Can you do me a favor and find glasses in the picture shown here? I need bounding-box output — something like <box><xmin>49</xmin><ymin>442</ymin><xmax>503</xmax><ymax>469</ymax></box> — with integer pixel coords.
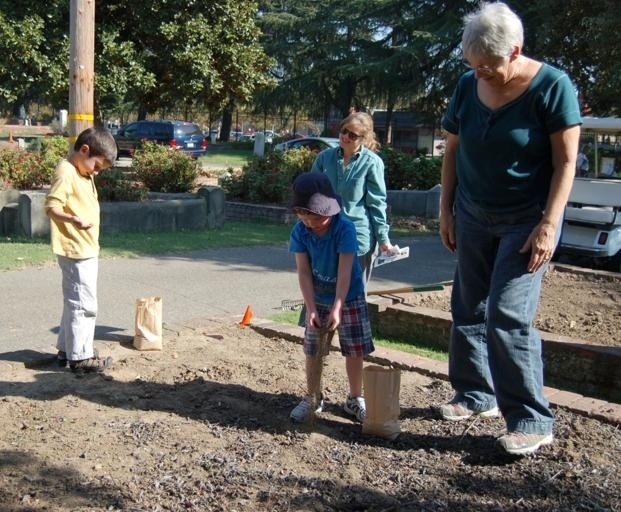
<box><xmin>339</xmin><ymin>127</ymin><xmax>364</xmax><ymax>141</ymax></box>
<box><xmin>461</xmin><ymin>56</ymin><xmax>504</xmax><ymax>74</ymax></box>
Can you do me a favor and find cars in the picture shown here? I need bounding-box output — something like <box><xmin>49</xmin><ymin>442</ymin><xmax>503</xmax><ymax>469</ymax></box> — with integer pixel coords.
<box><xmin>274</xmin><ymin>135</ymin><xmax>339</xmax><ymax>157</ymax></box>
<box><xmin>201</xmin><ymin>127</ymin><xmax>308</xmax><ymax>144</ymax></box>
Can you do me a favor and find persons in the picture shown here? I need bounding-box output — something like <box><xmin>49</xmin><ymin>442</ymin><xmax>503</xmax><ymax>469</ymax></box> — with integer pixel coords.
<box><xmin>438</xmin><ymin>3</ymin><xmax>584</xmax><ymax>458</ymax></box>
<box><xmin>289</xmin><ymin>172</ymin><xmax>375</xmax><ymax>422</ymax></box>
<box><xmin>297</xmin><ymin>112</ymin><xmax>395</xmax><ymax>366</ymax></box>
<box><xmin>45</xmin><ymin>126</ymin><xmax>118</xmax><ymax>373</ymax></box>
<box><xmin>576</xmin><ymin>142</ymin><xmax>592</xmax><ymax>177</ymax></box>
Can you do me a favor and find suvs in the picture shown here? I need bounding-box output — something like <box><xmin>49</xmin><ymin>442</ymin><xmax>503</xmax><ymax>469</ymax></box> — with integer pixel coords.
<box><xmin>112</xmin><ymin>117</ymin><xmax>207</xmax><ymax>164</ymax></box>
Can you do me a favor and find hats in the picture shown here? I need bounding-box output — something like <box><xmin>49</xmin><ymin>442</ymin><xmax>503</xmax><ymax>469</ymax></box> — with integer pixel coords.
<box><xmin>288</xmin><ymin>173</ymin><xmax>341</xmax><ymax>217</ymax></box>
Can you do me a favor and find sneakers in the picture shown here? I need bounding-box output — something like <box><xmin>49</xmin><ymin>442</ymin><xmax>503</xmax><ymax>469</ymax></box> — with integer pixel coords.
<box><xmin>437</xmin><ymin>400</ymin><xmax>501</xmax><ymax>421</ymax></box>
<box><xmin>344</xmin><ymin>396</ymin><xmax>365</xmax><ymax>422</ymax></box>
<box><xmin>290</xmin><ymin>392</ymin><xmax>323</xmax><ymax>423</ymax></box>
<box><xmin>494</xmin><ymin>431</ymin><xmax>553</xmax><ymax>455</ymax></box>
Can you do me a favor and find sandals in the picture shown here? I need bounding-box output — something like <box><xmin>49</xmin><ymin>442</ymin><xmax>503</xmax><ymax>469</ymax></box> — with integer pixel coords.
<box><xmin>69</xmin><ymin>356</ymin><xmax>112</xmax><ymax>372</ymax></box>
<box><xmin>57</xmin><ymin>348</ymin><xmax>99</xmax><ymax>367</ymax></box>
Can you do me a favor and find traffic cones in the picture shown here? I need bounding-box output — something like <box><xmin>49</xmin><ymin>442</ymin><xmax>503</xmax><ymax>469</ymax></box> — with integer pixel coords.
<box><xmin>238</xmin><ymin>305</ymin><xmax>255</xmax><ymax>326</ymax></box>
